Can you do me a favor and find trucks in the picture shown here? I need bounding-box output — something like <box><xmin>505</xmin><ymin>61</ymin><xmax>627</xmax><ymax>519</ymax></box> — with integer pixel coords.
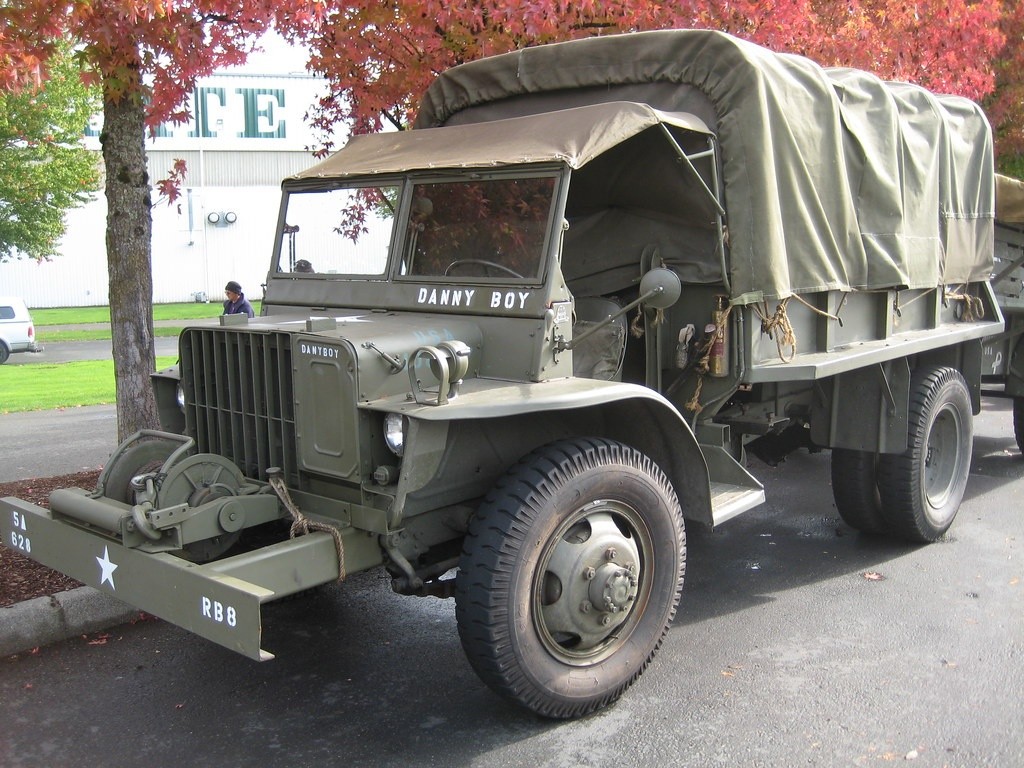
<box><xmin>0</xmin><ymin>27</ymin><xmax>1008</xmax><ymax>722</ymax></box>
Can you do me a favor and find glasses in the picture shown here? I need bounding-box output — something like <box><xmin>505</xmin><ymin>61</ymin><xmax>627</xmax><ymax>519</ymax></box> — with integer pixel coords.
<box><xmin>225</xmin><ymin>291</ymin><xmax>234</xmax><ymax>294</ymax></box>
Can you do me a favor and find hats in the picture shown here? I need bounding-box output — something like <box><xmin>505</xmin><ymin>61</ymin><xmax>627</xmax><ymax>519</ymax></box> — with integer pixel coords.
<box><xmin>225</xmin><ymin>281</ymin><xmax>242</xmax><ymax>294</ymax></box>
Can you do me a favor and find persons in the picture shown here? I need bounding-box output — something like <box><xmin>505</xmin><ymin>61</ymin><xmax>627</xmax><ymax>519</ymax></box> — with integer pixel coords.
<box><xmin>222</xmin><ymin>281</ymin><xmax>255</xmax><ymax>319</ymax></box>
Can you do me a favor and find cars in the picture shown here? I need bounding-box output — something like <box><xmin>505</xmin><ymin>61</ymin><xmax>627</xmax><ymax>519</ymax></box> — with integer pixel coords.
<box><xmin>0</xmin><ymin>303</ymin><xmax>46</xmax><ymax>363</ymax></box>
